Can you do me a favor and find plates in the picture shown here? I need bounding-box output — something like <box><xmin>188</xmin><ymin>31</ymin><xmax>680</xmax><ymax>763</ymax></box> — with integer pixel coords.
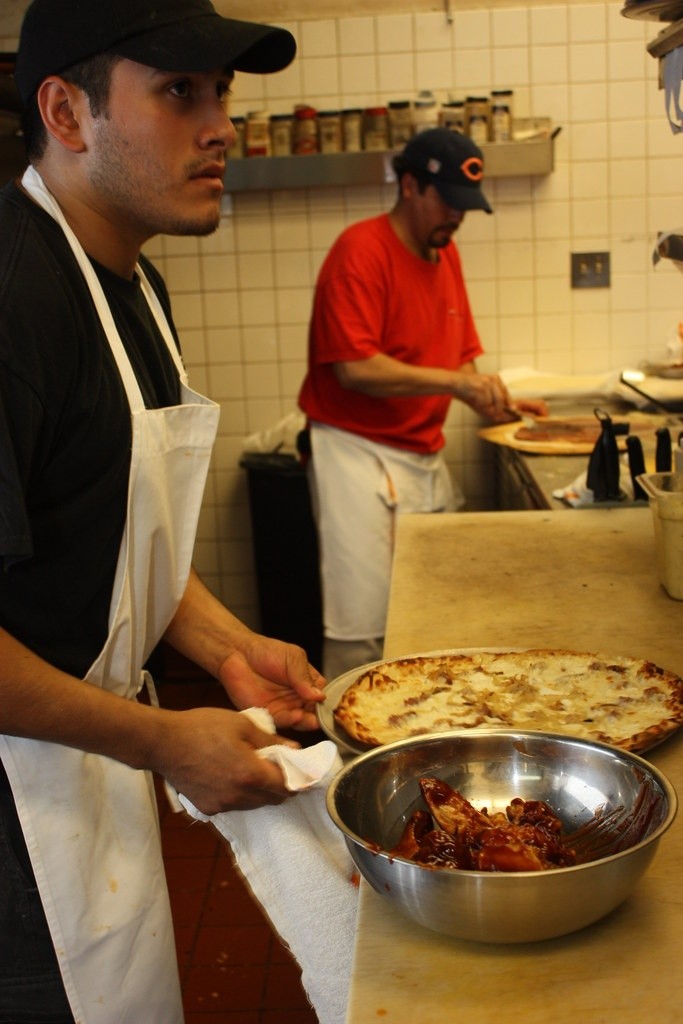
<box><xmin>506</xmin><ymin>426</ymin><xmax>601</xmax><ymax>448</ymax></box>
<box><xmin>314</xmin><ymin>646</ymin><xmax>675</xmax><ymax>757</ymax></box>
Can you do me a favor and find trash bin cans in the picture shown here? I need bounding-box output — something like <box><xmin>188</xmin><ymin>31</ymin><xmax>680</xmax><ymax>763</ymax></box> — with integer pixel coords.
<box><xmin>239</xmin><ymin>452</ymin><xmax>323</xmax><ymax>679</ymax></box>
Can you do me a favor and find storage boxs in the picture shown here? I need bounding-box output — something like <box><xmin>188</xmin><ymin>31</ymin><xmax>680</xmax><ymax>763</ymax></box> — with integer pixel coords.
<box><xmin>634</xmin><ymin>467</ymin><xmax>682</xmax><ymax>604</ymax></box>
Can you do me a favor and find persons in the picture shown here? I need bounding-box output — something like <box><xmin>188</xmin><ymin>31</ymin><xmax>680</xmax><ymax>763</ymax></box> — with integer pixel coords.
<box><xmin>298</xmin><ymin>129</ymin><xmax>524</xmax><ymax>683</ymax></box>
<box><xmin>0</xmin><ymin>0</ymin><xmax>328</xmax><ymax>1024</ymax></box>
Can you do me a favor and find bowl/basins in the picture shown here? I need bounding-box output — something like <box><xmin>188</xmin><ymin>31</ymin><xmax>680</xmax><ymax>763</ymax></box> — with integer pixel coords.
<box><xmin>327</xmin><ymin>728</ymin><xmax>679</xmax><ymax>945</ymax></box>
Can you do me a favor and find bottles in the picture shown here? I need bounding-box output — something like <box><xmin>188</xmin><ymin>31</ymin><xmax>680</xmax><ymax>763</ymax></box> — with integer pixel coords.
<box><xmin>222</xmin><ymin>88</ymin><xmax>516</xmax><ymax>159</ymax></box>
<box><xmin>586</xmin><ymin>408</ymin><xmax>620</xmax><ymax>503</ymax></box>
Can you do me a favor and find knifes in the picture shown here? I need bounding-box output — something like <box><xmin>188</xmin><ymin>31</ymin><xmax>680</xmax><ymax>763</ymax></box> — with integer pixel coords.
<box><xmin>626</xmin><ymin>428</ymin><xmax>673</xmax><ymax>502</ymax></box>
<box><xmin>504</xmin><ymin>406</ymin><xmax>540</xmax><ymax>432</ymax></box>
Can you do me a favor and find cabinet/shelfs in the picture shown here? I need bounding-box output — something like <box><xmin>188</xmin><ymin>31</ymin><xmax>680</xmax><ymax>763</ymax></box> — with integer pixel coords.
<box><xmin>496</xmin><ymin>448</ymin><xmax>537</xmax><ymax>511</ymax></box>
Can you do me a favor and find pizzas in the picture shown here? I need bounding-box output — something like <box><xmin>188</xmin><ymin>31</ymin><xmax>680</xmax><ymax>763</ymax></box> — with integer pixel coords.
<box><xmin>505</xmin><ymin>419</ymin><xmax>656</xmax><ymax>448</ymax></box>
<box><xmin>333</xmin><ymin>647</ymin><xmax>683</xmax><ymax>753</ymax></box>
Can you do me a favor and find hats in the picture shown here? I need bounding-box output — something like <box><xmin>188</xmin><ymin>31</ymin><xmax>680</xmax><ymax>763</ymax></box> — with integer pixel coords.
<box><xmin>14</xmin><ymin>0</ymin><xmax>297</xmax><ymax>110</ymax></box>
<box><xmin>401</xmin><ymin>127</ymin><xmax>493</xmax><ymax>216</ymax></box>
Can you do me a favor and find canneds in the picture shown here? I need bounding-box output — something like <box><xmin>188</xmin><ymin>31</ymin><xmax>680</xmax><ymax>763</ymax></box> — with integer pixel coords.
<box><xmin>226</xmin><ymin>89</ymin><xmax>514</xmax><ymax>161</ymax></box>
